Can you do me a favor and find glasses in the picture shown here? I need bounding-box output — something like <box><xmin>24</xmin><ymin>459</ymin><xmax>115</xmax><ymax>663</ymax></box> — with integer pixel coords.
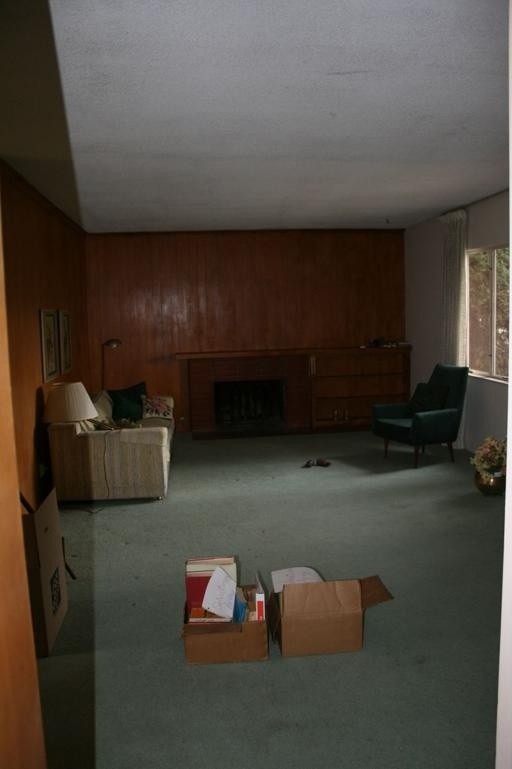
<box><xmin>41</xmin><ymin>382</ymin><xmax>120</xmax><ymax>430</ymax></box>
<box><xmin>101</xmin><ymin>338</ymin><xmax>122</xmax><ymax>390</ymax></box>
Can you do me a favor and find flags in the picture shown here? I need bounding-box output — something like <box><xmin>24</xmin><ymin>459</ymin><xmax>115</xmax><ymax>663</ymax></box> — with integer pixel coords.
<box><xmin>475</xmin><ymin>471</ymin><xmax>506</xmax><ymax>496</ymax></box>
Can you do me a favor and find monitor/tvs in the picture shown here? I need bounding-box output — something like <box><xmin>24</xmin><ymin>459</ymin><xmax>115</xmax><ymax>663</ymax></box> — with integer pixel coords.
<box><xmin>184</xmin><ymin>555</ymin><xmax>266</xmax><ymax>625</ymax></box>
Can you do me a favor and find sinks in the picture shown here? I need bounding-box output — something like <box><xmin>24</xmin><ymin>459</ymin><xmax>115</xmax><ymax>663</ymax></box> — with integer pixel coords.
<box><xmin>46</xmin><ymin>390</ymin><xmax>176</xmax><ymax>507</ymax></box>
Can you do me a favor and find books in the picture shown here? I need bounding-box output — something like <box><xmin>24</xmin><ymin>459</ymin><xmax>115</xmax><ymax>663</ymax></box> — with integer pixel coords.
<box><xmin>21</xmin><ymin>485</ymin><xmax>67</xmax><ymax>659</ymax></box>
<box><xmin>181</xmin><ymin>568</ymin><xmax>394</xmax><ymax>665</ymax></box>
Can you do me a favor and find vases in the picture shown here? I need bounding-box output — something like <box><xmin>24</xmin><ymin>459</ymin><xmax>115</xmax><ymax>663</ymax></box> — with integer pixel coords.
<box><xmin>467</xmin><ymin>433</ymin><xmax>507</xmax><ymax>481</ymax></box>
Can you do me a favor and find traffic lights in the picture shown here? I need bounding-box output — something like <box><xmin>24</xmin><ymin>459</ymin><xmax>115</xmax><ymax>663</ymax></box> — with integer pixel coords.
<box><xmin>404</xmin><ymin>383</ymin><xmax>450</xmax><ymax>418</ymax></box>
<box><xmin>106</xmin><ymin>381</ymin><xmax>174</xmax><ymax>424</ymax></box>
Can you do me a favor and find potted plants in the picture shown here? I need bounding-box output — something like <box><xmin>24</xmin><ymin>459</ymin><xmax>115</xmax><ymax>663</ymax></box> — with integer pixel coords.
<box><xmin>38</xmin><ymin>307</ymin><xmax>73</xmax><ymax>385</ymax></box>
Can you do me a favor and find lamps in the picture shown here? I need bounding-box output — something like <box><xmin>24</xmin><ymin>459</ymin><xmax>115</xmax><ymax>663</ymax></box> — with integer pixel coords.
<box><xmin>372</xmin><ymin>361</ymin><xmax>470</xmax><ymax>467</ymax></box>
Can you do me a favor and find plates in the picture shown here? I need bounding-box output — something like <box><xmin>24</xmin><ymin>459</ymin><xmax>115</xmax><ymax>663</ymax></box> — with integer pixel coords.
<box><xmin>308</xmin><ymin>344</ymin><xmax>412</xmax><ymax>434</ymax></box>
